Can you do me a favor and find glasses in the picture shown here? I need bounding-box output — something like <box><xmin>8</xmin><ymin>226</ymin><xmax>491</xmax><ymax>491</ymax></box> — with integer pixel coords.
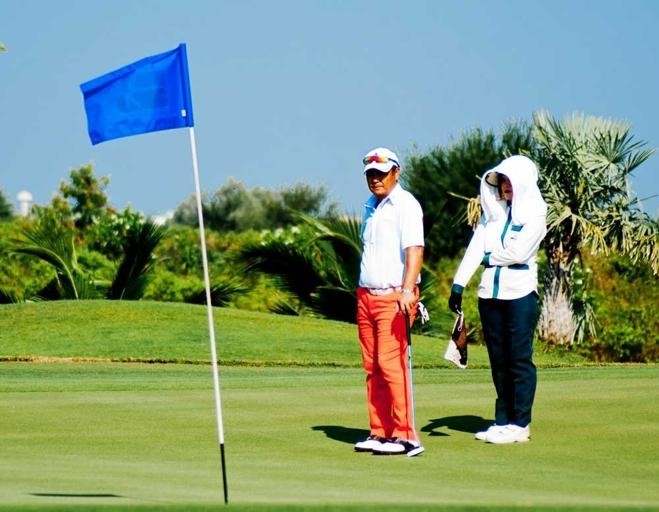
<box><xmin>363</xmin><ymin>156</ymin><xmax>400</xmax><ymax>167</ymax></box>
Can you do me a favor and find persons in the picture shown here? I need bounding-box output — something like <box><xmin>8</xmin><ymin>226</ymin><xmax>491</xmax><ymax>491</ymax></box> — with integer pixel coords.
<box><xmin>355</xmin><ymin>146</ymin><xmax>431</xmax><ymax>456</ymax></box>
<box><xmin>447</xmin><ymin>154</ymin><xmax>548</xmax><ymax>444</ymax></box>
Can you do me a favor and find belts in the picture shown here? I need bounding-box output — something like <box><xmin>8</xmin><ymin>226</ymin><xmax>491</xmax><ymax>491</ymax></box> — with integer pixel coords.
<box><xmin>366</xmin><ymin>287</ymin><xmax>403</xmax><ymax>296</ymax></box>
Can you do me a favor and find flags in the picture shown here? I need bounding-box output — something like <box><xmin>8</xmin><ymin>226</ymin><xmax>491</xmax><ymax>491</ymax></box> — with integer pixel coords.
<box><xmin>78</xmin><ymin>42</ymin><xmax>195</xmax><ymax>149</ymax></box>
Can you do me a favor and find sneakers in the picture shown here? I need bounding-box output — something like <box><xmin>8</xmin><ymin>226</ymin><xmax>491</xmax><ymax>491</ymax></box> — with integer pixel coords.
<box><xmin>474</xmin><ymin>424</ymin><xmax>529</xmax><ymax>444</ymax></box>
<box><xmin>371</xmin><ymin>437</ymin><xmax>419</xmax><ymax>454</ymax></box>
<box><xmin>354</xmin><ymin>435</ymin><xmax>389</xmax><ymax>452</ymax></box>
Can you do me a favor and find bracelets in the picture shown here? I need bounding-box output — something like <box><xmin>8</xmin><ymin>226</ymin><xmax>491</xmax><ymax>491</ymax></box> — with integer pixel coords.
<box><xmin>401</xmin><ymin>288</ymin><xmax>412</xmax><ymax>293</ymax></box>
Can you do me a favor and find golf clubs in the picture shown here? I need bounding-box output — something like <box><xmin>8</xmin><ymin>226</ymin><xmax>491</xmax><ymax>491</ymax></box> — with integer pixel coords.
<box><xmin>405</xmin><ymin>304</ymin><xmax>426</xmax><ymax>456</ymax></box>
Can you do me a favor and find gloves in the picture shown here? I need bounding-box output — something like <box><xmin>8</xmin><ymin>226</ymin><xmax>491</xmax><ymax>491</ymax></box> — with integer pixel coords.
<box><xmin>449</xmin><ymin>291</ymin><xmax>462</xmax><ymax>314</ymax></box>
<box><xmin>417</xmin><ymin>302</ymin><xmax>429</xmax><ymax>324</ymax></box>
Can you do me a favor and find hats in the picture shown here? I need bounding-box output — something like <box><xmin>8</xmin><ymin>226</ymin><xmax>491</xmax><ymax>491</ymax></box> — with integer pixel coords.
<box><xmin>363</xmin><ymin>147</ymin><xmax>400</xmax><ymax>173</ymax></box>
<box><xmin>485</xmin><ymin>172</ymin><xmax>500</xmax><ymax>187</ymax></box>
